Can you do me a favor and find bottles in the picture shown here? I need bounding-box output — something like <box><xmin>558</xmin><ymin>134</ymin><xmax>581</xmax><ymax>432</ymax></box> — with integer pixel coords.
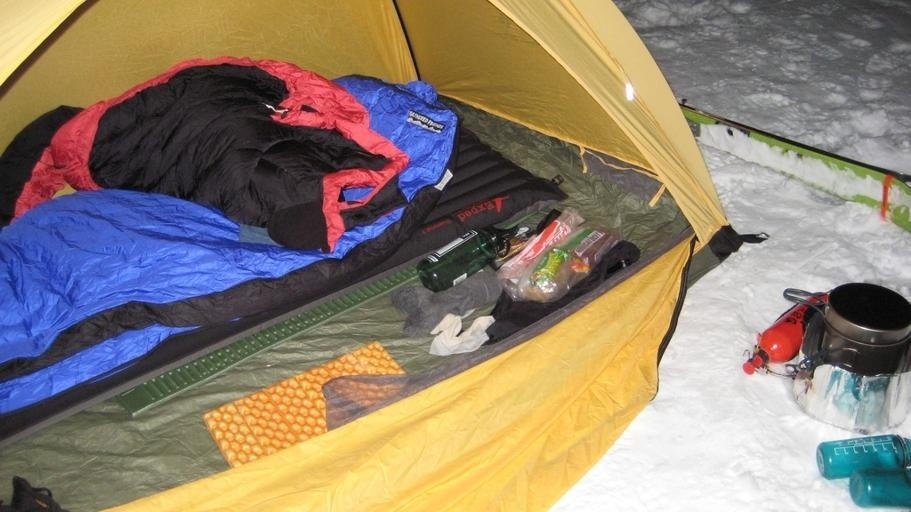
<box><xmin>849</xmin><ymin>467</ymin><xmax>911</xmax><ymax>510</ymax></box>
<box><xmin>416</xmin><ymin>224</ymin><xmax>520</xmax><ymax>292</ymax></box>
<box><xmin>815</xmin><ymin>433</ymin><xmax>911</xmax><ymax>480</ymax></box>
<box><xmin>743</xmin><ymin>291</ymin><xmax>831</xmax><ymax>375</ymax></box>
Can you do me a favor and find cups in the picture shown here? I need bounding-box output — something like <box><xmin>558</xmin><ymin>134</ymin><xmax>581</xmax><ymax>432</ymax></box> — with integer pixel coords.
<box><xmin>818</xmin><ymin>282</ymin><xmax>911</xmax><ymax>375</ymax></box>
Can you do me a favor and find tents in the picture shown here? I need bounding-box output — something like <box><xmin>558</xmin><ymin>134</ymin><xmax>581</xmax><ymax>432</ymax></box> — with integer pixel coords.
<box><xmin>1</xmin><ymin>0</ymin><xmax>769</xmax><ymax>512</ymax></box>
